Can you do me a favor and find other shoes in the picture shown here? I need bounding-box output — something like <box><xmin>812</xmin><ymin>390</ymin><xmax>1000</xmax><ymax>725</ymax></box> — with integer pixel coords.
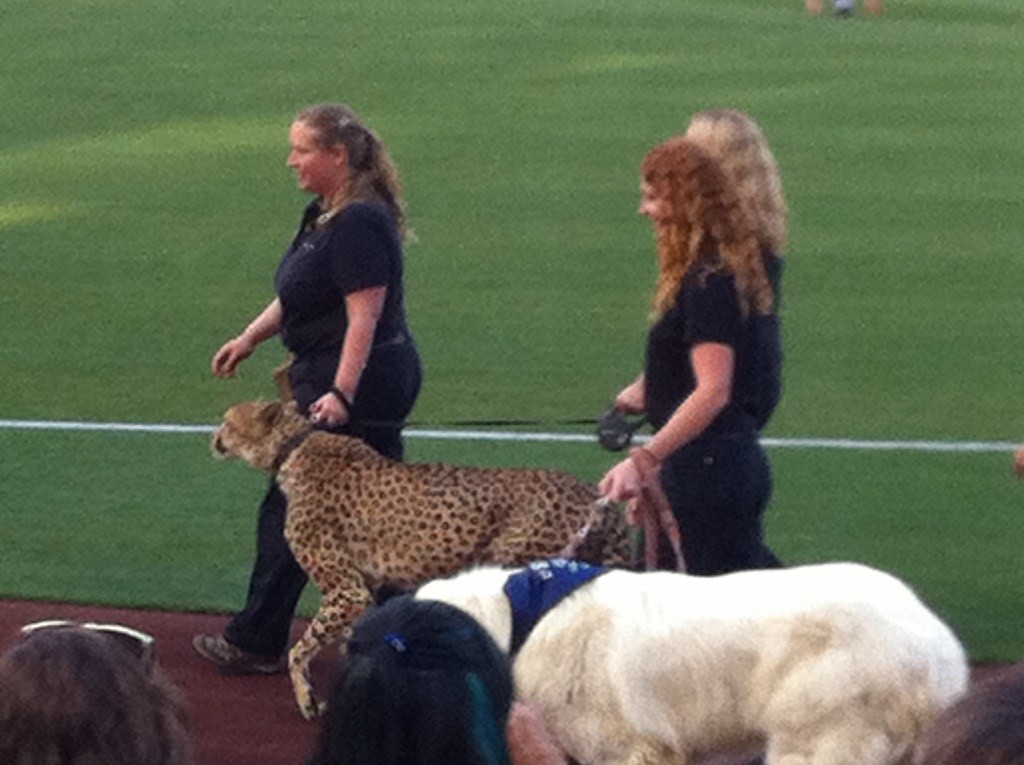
<box><xmin>193</xmin><ymin>630</ymin><xmax>286</xmax><ymax>677</ymax></box>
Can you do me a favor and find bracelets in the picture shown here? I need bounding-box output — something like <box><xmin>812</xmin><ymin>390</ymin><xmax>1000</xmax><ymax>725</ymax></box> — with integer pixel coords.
<box><xmin>642</xmin><ymin>447</ymin><xmax>655</xmax><ymax>465</ymax></box>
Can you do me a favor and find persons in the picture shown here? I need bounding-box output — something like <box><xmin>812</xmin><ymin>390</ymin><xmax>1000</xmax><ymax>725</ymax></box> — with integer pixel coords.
<box><xmin>614</xmin><ymin>108</ymin><xmax>789</xmax><ymax>432</ymax></box>
<box><xmin>0</xmin><ymin>617</ymin><xmax>192</xmax><ymax>765</ymax></box>
<box><xmin>597</xmin><ymin>138</ymin><xmax>782</xmax><ymax>575</ymax></box>
<box><xmin>192</xmin><ymin>104</ymin><xmax>423</xmax><ymax>676</ymax></box>
<box><xmin>312</xmin><ymin>599</ymin><xmax>566</xmax><ymax>764</ymax></box>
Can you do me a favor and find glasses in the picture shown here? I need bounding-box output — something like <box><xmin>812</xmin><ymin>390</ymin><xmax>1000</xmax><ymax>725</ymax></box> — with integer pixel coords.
<box><xmin>21</xmin><ymin>617</ymin><xmax>155</xmax><ymax>662</ymax></box>
